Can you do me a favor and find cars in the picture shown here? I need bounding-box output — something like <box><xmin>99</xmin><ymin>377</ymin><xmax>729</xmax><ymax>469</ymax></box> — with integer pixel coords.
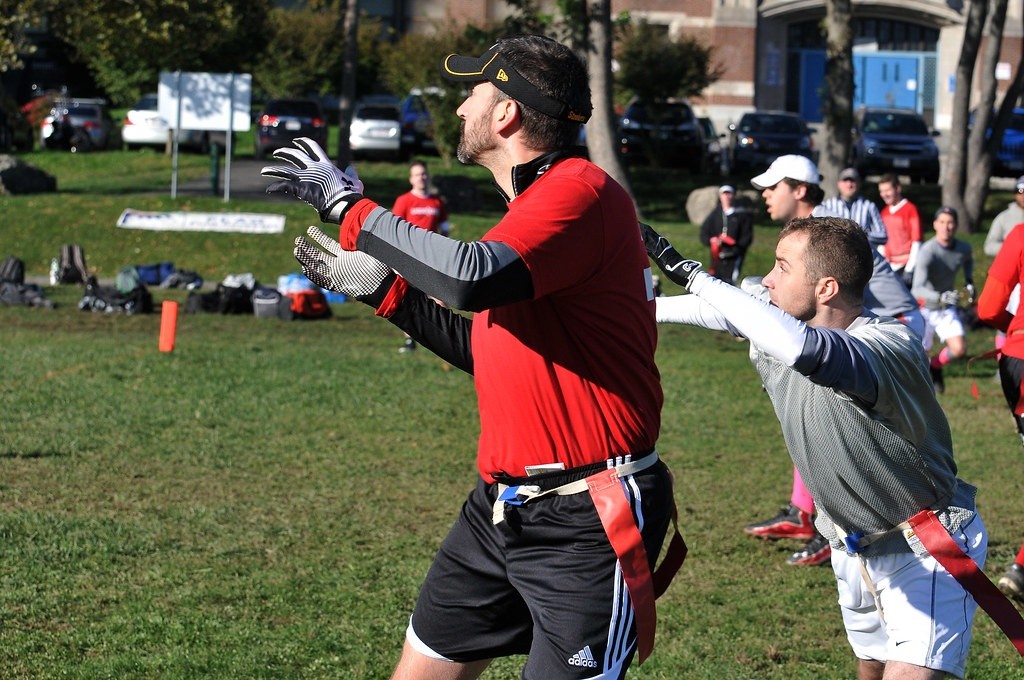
<box><xmin>726</xmin><ymin>110</ymin><xmax>817</xmax><ymax>182</ymax></box>
<box><xmin>121</xmin><ymin>95</ymin><xmax>238</xmax><ymax>155</ymax></box>
<box><xmin>843</xmin><ymin>106</ymin><xmax>941</xmax><ymax>186</ymax></box>
<box><xmin>40</xmin><ymin>98</ymin><xmax>110</xmax><ymax>152</ymax></box>
<box><xmin>618</xmin><ymin>97</ymin><xmax>725</xmax><ymax>170</ymax></box>
<box><xmin>969</xmin><ymin>106</ymin><xmax>1024</xmax><ymax>179</ymax></box>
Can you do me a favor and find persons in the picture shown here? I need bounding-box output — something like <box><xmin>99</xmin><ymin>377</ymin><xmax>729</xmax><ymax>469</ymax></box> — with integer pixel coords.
<box><xmin>742</xmin><ymin>154</ymin><xmax>926</xmax><ymax>567</ymax></box>
<box><xmin>909</xmin><ymin>207</ymin><xmax>976</xmax><ymax>394</ymax></box>
<box><xmin>639</xmin><ymin>217</ymin><xmax>989</xmax><ymax>680</ymax></box>
<box><xmin>820</xmin><ymin>167</ymin><xmax>887</xmax><ymax>246</ymax></box>
<box><xmin>391</xmin><ymin>161</ymin><xmax>449</xmax><ymax>353</ymax></box>
<box><xmin>877</xmin><ymin>173</ymin><xmax>922</xmax><ymax>289</ymax></box>
<box><xmin>698</xmin><ymin>182</ymin><xmax>754</xmax><ymax>286</ymax></box>
<box><xmin>977</xmin><ymin>175</ymin><xmax>1024</xmax><ymax>608</ymax></box>
<box><xmin>262</xmin><ymin>36</ymin><xmax>687</xmax><ymax>680</ymax></box>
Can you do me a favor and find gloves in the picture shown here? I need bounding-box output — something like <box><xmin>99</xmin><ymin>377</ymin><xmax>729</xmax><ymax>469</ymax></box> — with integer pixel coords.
<box><xmin>293</xmin><ymin>225</ymin><xmax>396</xmax><ymax>309</ymax></box>
<box><xmin>939</xmin><ymin>289</ymin><xmax>960</xmax><ymax>306</ymax></box>
<box><xmin>638</xmin><ymin>221</ymin><xmax>703</xmax><ymax>291</ymax></box>
<box><xmin>965</xmin><ymin>282</ymin><xmax>977</xmax><ymax>303</ymax></box>
<box><xmin>260</xmin><ymin>137</ymin><xmax>364</xmax><ymax>223</ymax></box>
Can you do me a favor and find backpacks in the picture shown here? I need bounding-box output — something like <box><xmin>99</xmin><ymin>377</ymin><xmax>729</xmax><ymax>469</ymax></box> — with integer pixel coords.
<box><xmin>1</xmin><ymin>254</ymin><xmax>25</xmax><ymax>284</ymax></box>
<box><xmin>49</xmin><ymin>243</ymin><xmax>88</xmax><ymax>286</ymax></box>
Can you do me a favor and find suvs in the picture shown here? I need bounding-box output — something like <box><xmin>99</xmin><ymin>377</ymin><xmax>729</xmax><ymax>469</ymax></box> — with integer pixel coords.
<box><xmin>254</xmin><ymin>87</ymin><xmax>467</xmax><ymax>163</ymax></box>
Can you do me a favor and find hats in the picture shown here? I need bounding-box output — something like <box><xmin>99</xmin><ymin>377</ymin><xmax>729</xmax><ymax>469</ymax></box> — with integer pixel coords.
<box><xmin>719</xmin><ymin>184</ymin><xmax>736</xmax><ymax>194</ymax></box>
<box><xmin>439</xmin><ymin>42</ymin><xmax>594</xmax><ymax>124</ymax></box>
<box><xmin>1013</xmin><ymin>175</ymin><xmax>1024</xmax><ymax>194</ymax></box>
<box><xmin>934</xmin><ymin>206</ymin><xmax>958</xmax><ymax>225</ymax></box>
<box><xmin>838</xmin><ymin>167</ymin><xmax>861</xmax><ymax>182</ymax></box>
<box><xmin>750</xmin><ymin>154</ymin><xmax>821</xmax><ymax>191</ymax></box>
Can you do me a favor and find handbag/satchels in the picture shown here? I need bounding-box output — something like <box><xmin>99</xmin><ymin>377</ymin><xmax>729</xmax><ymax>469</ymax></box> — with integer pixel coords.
<box><xmin>78</xmin><ymin>262</ymin><xmax>349</xmax><ymax>321</ymax></box>
<box><xmin>0</xmin><ymin>284</ymin><xmax>58</xmax><ymax>310</ymax></box>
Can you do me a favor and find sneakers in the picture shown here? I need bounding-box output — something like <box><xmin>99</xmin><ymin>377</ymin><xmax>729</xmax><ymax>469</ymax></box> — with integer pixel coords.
<box><xmin>783</xmin><ymin>532</ymin><xmax>831</xmax><ymax>567</ymax></box>
<box><xmin>742</xmin><ymin>504</ymin><xmax>814</xmax><ymax>542</ymax></box>
<box><xmin>930</xmin><ymin>365</ymin><xmax>945</xmax><ymax>394</ymax></box>
<box><xmin>399</xmin><ymin>337</ymin><xmax>416</xmax><ymax>352</ymax></box>
